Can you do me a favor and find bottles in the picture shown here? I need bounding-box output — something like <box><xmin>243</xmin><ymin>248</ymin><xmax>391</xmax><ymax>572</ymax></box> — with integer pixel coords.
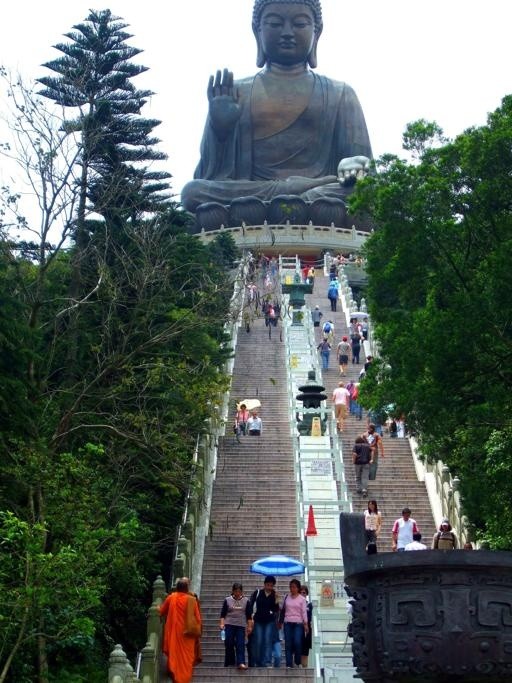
<box><xmin>221</xmin><ymin>629</ymin><xmax>226</xmax><ymax>641</ymax></box>
<box><xmin>279</xmin><ymin>628</ymin><xmax>283</xmax><ymax>640</ymax></box>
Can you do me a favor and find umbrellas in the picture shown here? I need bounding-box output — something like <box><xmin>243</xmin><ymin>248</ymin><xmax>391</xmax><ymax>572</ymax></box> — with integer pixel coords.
<box><xmin>235</xmin><ymin>397</ymin><xmax>263</xmax><ymax>412</ymax></box>
<box><xmin>350</xmin><ymin>310</ymin><xmax>370</xmax><ymax>320</ymax></box>
<box><xmin>248</xmin><ymin>554</ymin><xmax>306</xmax><ymax>604</ymax></box>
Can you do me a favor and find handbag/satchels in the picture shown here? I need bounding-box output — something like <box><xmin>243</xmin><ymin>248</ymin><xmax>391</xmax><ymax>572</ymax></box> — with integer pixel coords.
<box><xmin>250</xmin><ymin>590</ymin><xmax>261</xmax><ymax>617</ymax></box>
<box><xmin>184</xmin><ymin>595</ymin><xmax>201</xmax><ymax>636</ymax></box>
<box><xmin>279</xmin><ymin>594</ymin><xmax>288</xmax><ymax>623</ymax></box>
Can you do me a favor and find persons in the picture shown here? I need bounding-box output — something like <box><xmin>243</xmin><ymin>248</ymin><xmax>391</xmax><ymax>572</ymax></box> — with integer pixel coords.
<box><xmin>404</xmin><ymin>531</ymin><xmax>427</xmax><ymax>551</ymax></box>
<box><xmin>321</xmin><ymin>319</ymin><xmax>335</xmax><ymax>349</ymax></box>
<box><xmin>364</xmin><ymin>355</ymin><xmax>374</xmax><ymax>373</ymax></box>
<box><xmin>179</xmin><ymin>0</ymin><xmax>408</xmax><ymax>243</ymax></box>
<box><xmin>352</xmin><ymin>382</ymin><xmax>363</xmax><ymax>420</ymax></box>
<box><xmin>336</xmin><ymin>336</ymin><xmax>351</xmax><ymax>378</ymax></box>
<box><xmin>277</xmin><ymin>579</ymin><xmax>309</xmax><ymax>668</ymax></box>
<box><xmin>302</xmin><ymin>264</ymin><xmax>310</xmax><ymax>286</ymax></box>
<box><xmin>329</xmin><ymin>264</ymin><xmax>339</xmax><ymax>282</ymax></box>
<box><xmin>219</xmin><ymin>583</ymin><xmax>253</xmax><ymax>670</ymax></box>
<box><xmin>156</xmin><ymin>576</ymin><xmax>202</xmax><ymax>683</ymax></box>
<box><xmin>362</xmin><ymin>317</ymin><xmax>370</xmax><ymax>341</ymax></box>
<box><xmin>246</xmin><ymin>410</ymin><xmax>262</xmax><ymax>436</ymax></box>
<box><xmin>299</xmin><ymin>585</ymin><xmax>313</xmax><ymax>668</ymax></box>
<box><xmin>391</xmin><ymin>508</ymin><xmax>417</xmax><ymax>552</ymax></box>
<box><xmin>429</xmin><ymin>519</ymin><xmax>459</xmax><ymax>552</ymax></box>
<box><xmin>363</xmin><ymin>423</ymin><xmax>384</xmax><ymax>479</ymax></box>
<box><xmin>346</xmin><ymin>380</ymin><xmax>358</xmax><ymax>413</ymax></box>
<box><xmin>328</xmin><ymin>284</ymin><xmax>339</xmax><ymax>312</ymax></box>
<box><xmin>234</xmin><ymin>404</ymin><xmax>249</xmax><ymax>436</ymax></box>
<box><xmin>365</xmin><ymin>406</ymin><xmax>387</xmax><ymax>438</ymax></box>
<box><xmin>348</xmin><ymin>330</ymin><xmax>365</xmax><ymax>365</ymax></box>
<box><xmin>362</xmin><ymin>500</ymin><xmax>382</xmax><ymax>545</ymax></box>
<box><xmin>348</xmin><ymin>318</ymin><xmax>359</xmax><ymax>333</ymax></box>
<box><xmin>306</xmin><ymin>267</ymin><xmax>315</xmax><ymax>285</ymax></box>
<box><xmin>249</xmin><ymin>576</ymin><xmax>280</xmax><ymax>667</ymax></box>
<box><xmin>351</xmin><ymin>436</ymin><xmax>374</xmax><ymax>498</ymax></box>
<box><xmin>247</xmin><ymin>249</ymin><xmax>278</xmax><ymax>298</ymax></box>
<box><xmin>329</xmin><ymin>278</ymin><xmax>339</xmax><ymax>290</ymax></box>
<box><xmin>272</xmin><ymin>628</ymin><xmax>282</xmax><ymax>668</ymax></box>
<box><xmin>310</xmin><ymin>304</ymin><xmax>323</xmax><ymax>327</ymax></box>
<box><xmin>316</xmin><ymin>337</ymin><xmax>332</xmax><ymax>372</ymax></box>
<box><xmin>331</xmin><ymin>381</ymin><xmax>351</xmax><ymax>434</ymax></box>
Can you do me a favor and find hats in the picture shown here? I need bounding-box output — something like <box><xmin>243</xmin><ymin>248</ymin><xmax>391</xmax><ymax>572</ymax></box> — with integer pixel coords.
<box><xmin>342</xmin><ymin>336</ymin><xmax>347</xmax><ymax>340</ymax></box>
<box><xmin>442</xmin><ymin>518</ymin><xmax>449</xmax><ymax>526</ymax></box>
